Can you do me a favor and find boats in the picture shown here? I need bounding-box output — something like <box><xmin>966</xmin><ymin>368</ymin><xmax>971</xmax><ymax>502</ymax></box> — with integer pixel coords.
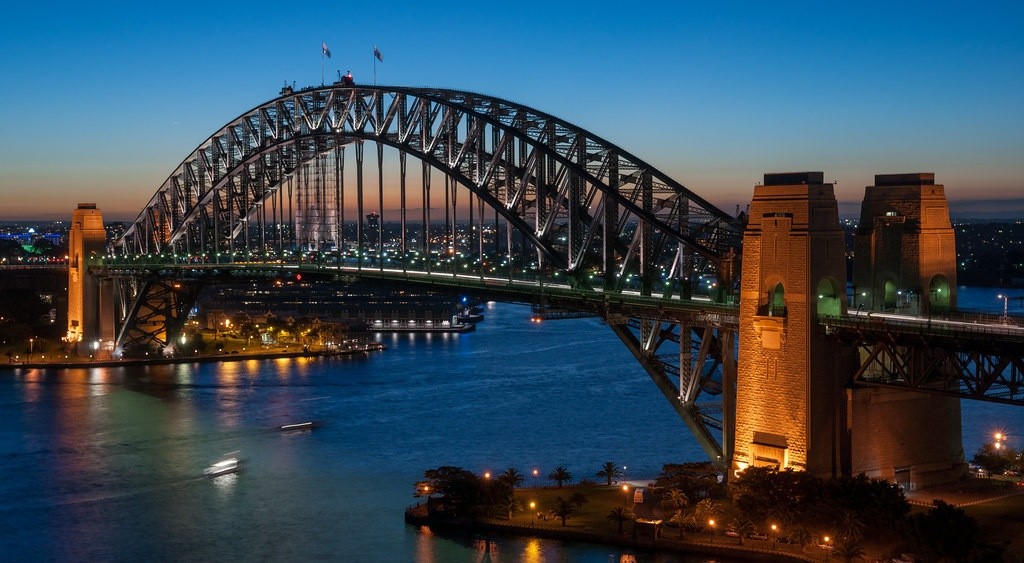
<box><xmin>203</xmin><ymin>455</ymin><xmax>247</xmax><ymax>476</ymax></box>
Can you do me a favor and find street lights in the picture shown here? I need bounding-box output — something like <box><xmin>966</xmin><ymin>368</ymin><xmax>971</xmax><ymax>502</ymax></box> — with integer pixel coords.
<box><xmin>530</xmin><ymin>500</ymin><xmax>537</xmax><ymax>527</ymax></box>
<box><xmin>997</xmin><ymin>293</ymin><xmax>1008</xmax><ymax>324</ymax></box>
<box><xmin>533</xmin><ymin>468</ymin><xmax>539</xmax><ymax>493</ymax></box>
<box><xmin>622</xmin><ymin>484</ymin><xmax>629</xmax><ymax>508</ymax></box>
<box><xmin>770</xmin><ymin>523</ymin><xmax>778</xmax><ymax>548</ymax></box>
<box><xmin>709</xmin><ymin>519</ymin><xmax>715</xmax><ymax>543</ymax></box>
<box><xmin>824</xmin><ymin>535</ymin><xmax>830</xmax><ymax>558</ymax></box>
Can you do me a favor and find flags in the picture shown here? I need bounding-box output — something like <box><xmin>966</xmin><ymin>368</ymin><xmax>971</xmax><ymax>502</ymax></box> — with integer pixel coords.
<box><xmin>322</xmin><ymin>43</ymin><xmax>330</xmax><ymax>59</ymax></box>
<box><xmin>374</xmin><ymin>47</ymin><xmax>383</xmax><ymax>62</ymax></box>
